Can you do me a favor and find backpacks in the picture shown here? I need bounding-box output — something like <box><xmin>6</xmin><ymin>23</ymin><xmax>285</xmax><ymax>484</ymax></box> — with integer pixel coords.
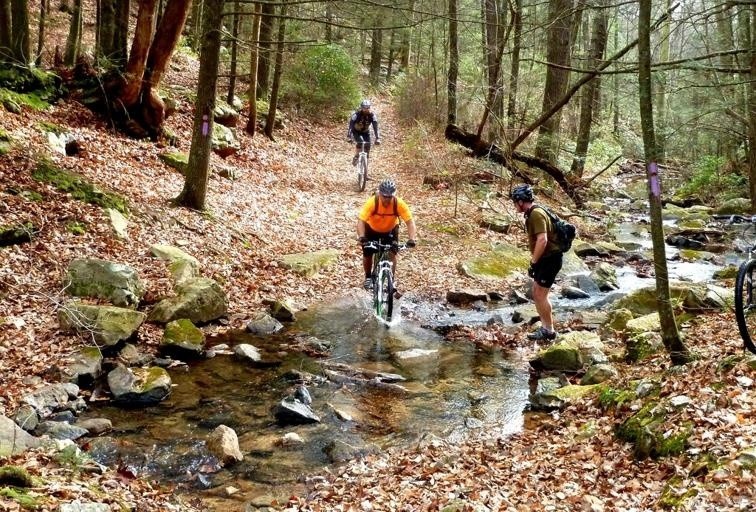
<box><xmin>528</xmin><ymin>205</ymin><xmax>574</xmax><ymax>252</ymax></box>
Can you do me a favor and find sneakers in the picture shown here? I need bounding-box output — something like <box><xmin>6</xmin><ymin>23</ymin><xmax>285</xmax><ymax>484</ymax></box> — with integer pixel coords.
<box><xmin>527</xmin><ymin>327</ymin><xmax>555</xmax><ymax>340</ymax></box>
<box><xmin>363</xmin><ymin>278</ymin><xmax>372</xmax><ymax>288</ymax></box>
<box><xmin>352</xmin><ymin>154</ymin><xmax>359</xmax><ymax>166</ymax></box>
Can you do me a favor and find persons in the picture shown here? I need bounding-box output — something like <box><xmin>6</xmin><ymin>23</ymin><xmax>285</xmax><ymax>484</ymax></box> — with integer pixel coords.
<box><xmin>355</xmin><ymin>179</ymin><xmax>419</xmax><ymax>289</ymax></box>
<box><xmin>512</xmin><ymin>185</ymin><xmax>576</xmax><ymax>341</ymax></box>
<box><xmin>347</xmin><ymin>99</ymin><xmax>381</xmax><ymax>164</ymax></box>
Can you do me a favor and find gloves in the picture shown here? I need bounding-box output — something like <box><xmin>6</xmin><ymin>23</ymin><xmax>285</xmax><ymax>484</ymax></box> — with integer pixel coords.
<box><xmin>527</xmin><ymin>261</ymin><xmax>535</xmax><ymax>278</ymax></box>
<box><xmin>404</xmin><ymin>239</ymin><xmax>414</xmax><ymax>247</ymax></box>
<box><xmin>358</xmin><ymin>237</ymin><xmax>367</xmax><ymax>243</ymax></box>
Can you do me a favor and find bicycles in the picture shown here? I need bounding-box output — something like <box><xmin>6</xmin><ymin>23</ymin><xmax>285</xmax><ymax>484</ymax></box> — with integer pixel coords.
<box><xmin>348</xmin><ymin>135</ymin><xmax>377</xmax><ymax>193</ymax></box>
<box><xmin>711</xmin><ymin>214</ymin><xmax>756</xmax><ymax>356</ymax></box>
<box><xmin>359</xmin><ymin>236</ymin><xmax>415</xmax><ymax>327</ymax></box>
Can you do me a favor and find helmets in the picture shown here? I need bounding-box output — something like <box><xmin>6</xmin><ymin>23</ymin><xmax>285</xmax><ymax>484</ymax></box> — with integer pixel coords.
<box><xmin>360</xmin><ymin>100</ymin><xmax>369</xmax><ymax>108</ymax></box>
<box><xmin>511</xmin><ymin>184</ymin><xmax>532</xmax><ymax>201</ymax></box>
<box><xmin>378</xmin><ymin>180</ymin><xmax>396</xmax><ymax>197</ymax></box>
